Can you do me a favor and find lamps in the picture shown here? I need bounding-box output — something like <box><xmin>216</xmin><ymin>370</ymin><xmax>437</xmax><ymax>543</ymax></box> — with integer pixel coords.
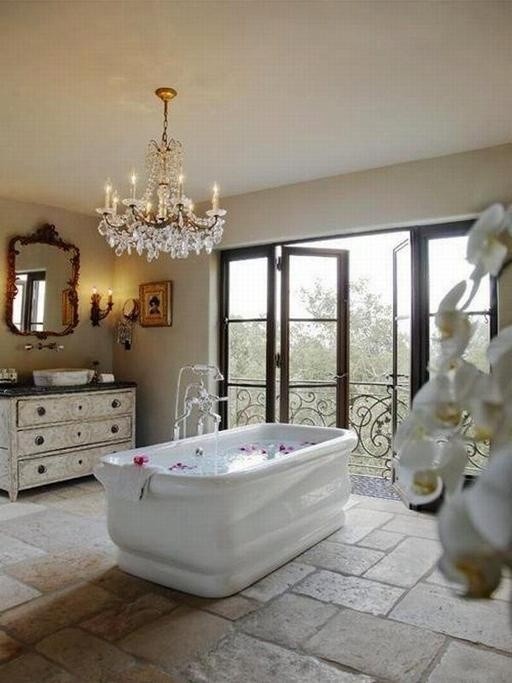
<box><xmin>94</xmin><ymin>86</ymin><xmax>228</xmax><ymax>263</ymax></box>
<box><xmin>90</xmin><ymin>285</ymin><xmax>115</xmax><ymax>326</ymax></box>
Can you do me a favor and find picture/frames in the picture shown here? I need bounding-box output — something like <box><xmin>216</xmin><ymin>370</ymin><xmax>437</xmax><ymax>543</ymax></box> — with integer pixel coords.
<box><xmin>139</xmin><ymin>279</ymin><xmax>173</xmax><ymax>328</ymax></box>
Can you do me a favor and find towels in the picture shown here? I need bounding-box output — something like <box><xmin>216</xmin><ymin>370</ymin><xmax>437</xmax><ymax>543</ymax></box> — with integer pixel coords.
<box><xmin>111</xmin><ymin>461</ymin><xmax>166</xmax><ymax>502</ymax></box>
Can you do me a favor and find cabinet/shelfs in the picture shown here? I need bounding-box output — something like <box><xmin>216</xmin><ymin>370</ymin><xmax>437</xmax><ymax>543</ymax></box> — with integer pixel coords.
<box><xmin>1</xmin><ymin>387</ymin><xmax>136</xmax><ymax>501</ymax></box>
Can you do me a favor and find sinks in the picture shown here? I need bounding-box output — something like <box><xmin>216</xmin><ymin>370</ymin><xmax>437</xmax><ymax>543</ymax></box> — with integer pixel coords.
<box><xmin>32</xmin><ymin>367</ymin><xmax>95</xmax><ymax>387</ymax></box>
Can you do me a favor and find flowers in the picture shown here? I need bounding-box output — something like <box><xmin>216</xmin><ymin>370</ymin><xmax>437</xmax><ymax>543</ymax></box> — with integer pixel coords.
<box><xmin>390</xmin><ymin>201</ymin><xmax>512</xmax><ymax>597</ymax></box>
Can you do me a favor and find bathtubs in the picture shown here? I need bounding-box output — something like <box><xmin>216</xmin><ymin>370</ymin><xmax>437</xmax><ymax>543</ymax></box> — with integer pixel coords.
<box><xmin>94</xmin><ymin>422</ymin><xmax>359</xmax><ymax>599</ymax></box>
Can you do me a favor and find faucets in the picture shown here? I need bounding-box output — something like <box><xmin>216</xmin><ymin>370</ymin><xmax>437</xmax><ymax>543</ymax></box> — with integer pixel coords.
<box><xmin>36</xmin><ymin>342</ymin><xmax>65</xmax><ymax>352</ymax></box>
<box><xmin>209</xmin><ymin>412</ymin><xmax>223</xmax><ymax>422</ymax></box>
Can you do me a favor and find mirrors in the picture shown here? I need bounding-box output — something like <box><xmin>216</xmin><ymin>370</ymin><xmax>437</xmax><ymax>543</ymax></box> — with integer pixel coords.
<box><xmin>6</xmin><ymin>222</ymin><xmax>81</xmax><ymax>341</ymax></box>
